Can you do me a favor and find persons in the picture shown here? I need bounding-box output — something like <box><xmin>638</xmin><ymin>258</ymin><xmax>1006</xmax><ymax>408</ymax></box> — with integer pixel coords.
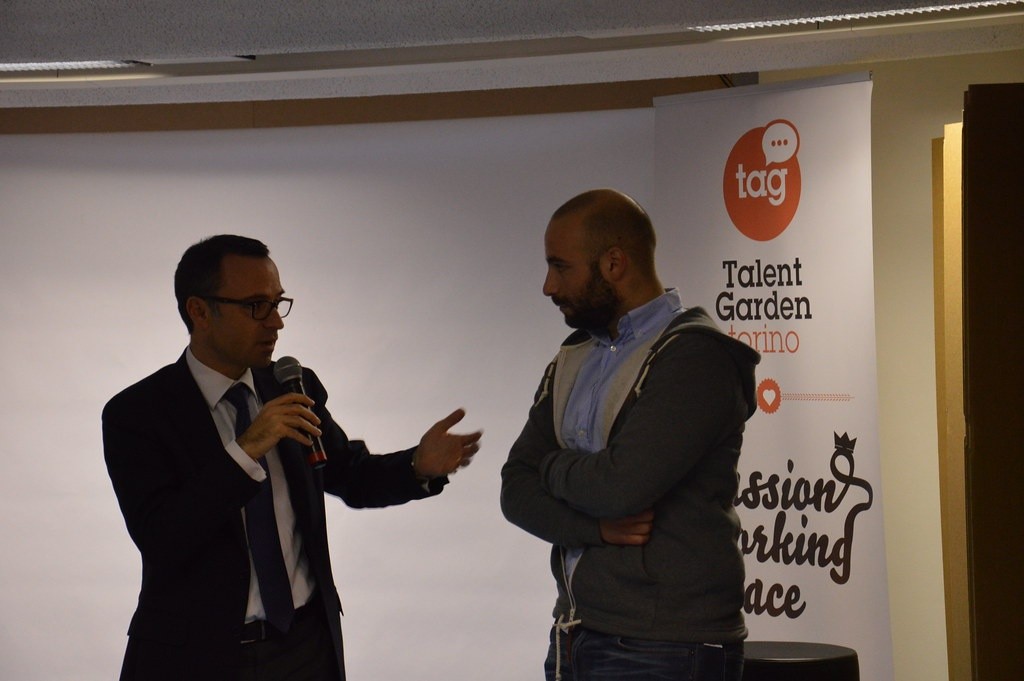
<box><xmin>102</xmin><ymin>235</ymin><xmax>484</xmax><ymax>680</ymax></box>
<box><xmin>499</xmin><ymin>190</ymin><xmax>760</xmax><ymax>680</ymax></box>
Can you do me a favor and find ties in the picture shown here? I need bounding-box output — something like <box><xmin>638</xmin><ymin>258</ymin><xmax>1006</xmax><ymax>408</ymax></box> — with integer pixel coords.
<box><xmin>223</xmin><ymin>382</ymin><xmax>294</xmax><ymax>634</ymax></box>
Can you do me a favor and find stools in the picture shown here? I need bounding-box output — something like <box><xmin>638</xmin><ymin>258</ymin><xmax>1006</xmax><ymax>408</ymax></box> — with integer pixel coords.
<box><xmin>741</xmin><ymin>642</ymin><xmax>861</xmax><ymax>681</ymax></box>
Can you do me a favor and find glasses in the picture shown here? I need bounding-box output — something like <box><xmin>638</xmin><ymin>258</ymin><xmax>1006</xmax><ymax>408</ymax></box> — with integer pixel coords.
<box><xmin>203</xmin><ymin>297</ymin><xmax>294</xmax><ymax>321</ymax></box>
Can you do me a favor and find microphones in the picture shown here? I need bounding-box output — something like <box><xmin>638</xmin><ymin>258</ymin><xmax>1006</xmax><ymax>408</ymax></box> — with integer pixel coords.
<box><xmin>273</xmin><ymin>356</ymin><xmax>328</xmax><ymax>471</ymax></box>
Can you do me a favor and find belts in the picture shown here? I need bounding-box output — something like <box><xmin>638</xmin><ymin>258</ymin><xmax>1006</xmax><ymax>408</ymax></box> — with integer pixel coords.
<box><xmin>240</xmin><ymin>595</ymin><xmax>318</xmax><ymax>645</ymax></box>
